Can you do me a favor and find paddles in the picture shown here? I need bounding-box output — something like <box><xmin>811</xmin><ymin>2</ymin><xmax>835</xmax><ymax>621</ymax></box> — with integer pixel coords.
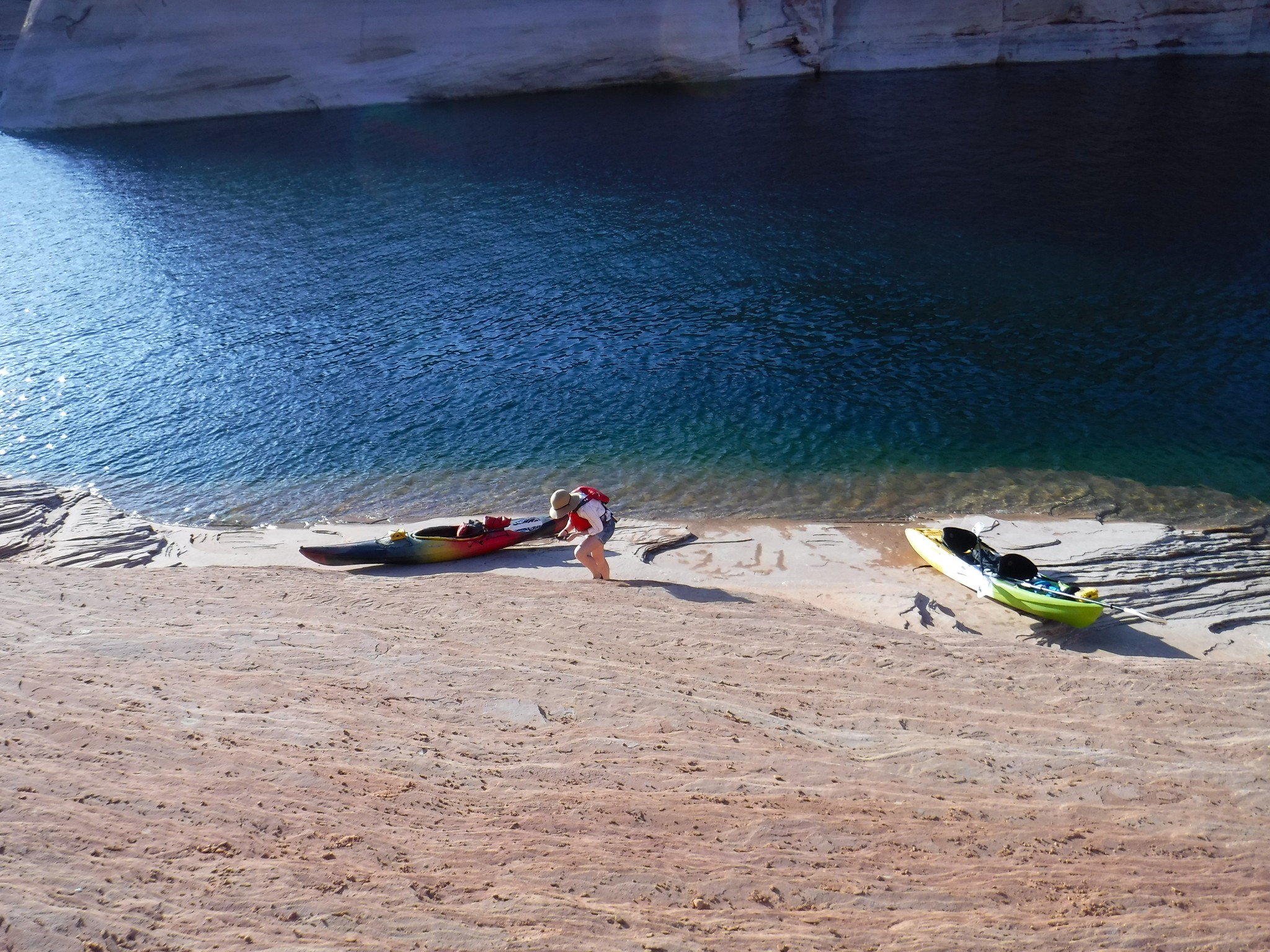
<box><xmin>971</xmin><ymin>520</ymin><xmax>990</xmax><ymax>599</ymax></box>
<box><xmin>983</xmin><ymin>568</ymin><xmax>1167</xmax><ymax>626</ymax></box>
<box><xmin>452</xmin><ymin>517</ymin><xmax>544</xmax><ymax>539</ymax></box>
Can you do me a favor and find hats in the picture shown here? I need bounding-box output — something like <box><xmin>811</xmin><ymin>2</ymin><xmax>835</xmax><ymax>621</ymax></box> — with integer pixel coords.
<box><xmin>549</xmin><ymin>489</ymin><xmax>579</xmax><ymax>519</ymax></box>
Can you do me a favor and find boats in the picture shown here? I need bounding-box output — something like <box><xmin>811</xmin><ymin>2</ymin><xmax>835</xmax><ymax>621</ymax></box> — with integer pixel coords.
<box><xmin>903</xmin><ymin>527</ymin><xmax>1106</xmax><ymax>630</ymax></box>
<box><xmin>299</xmin><ymin>516</ymin><xmax>554</xmax><ymax>568</ymax></box>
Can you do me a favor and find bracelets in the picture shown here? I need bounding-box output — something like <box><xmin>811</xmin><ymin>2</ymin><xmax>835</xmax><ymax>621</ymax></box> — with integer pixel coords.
<box><xmin>563</xmin><ymin>528</ymin><xmax>568</xmax><ymax>535</ymax></box>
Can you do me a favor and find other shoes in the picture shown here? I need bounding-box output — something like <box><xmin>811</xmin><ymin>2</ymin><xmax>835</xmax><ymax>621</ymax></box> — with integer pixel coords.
<box><xmin>593</xmin><ymin>573</ymin><xmax>603</xmax><ymax>579</ymax></box>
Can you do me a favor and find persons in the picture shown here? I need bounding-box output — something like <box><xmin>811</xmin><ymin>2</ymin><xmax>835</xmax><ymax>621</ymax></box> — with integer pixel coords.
<box><xmin>549</xmin><ymin>486</ymin><xmax>615</xmax><ymax>581</ymax></box>
